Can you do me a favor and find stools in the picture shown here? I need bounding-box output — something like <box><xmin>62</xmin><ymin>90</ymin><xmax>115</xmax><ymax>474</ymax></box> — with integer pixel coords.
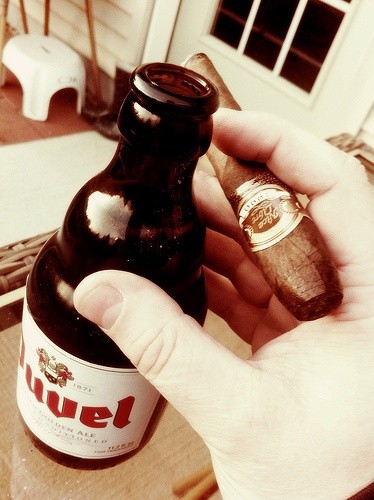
<box><xmin>2</xmin><ymin>32</ymin><xmax>86</xmax><ymax>122</ymax></box>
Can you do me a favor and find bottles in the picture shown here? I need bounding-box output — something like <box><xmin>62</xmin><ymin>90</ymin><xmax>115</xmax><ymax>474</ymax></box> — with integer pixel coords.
<box><xmin>15</xmin><ymin>62</ymin><xmax>221</xmax><ymax>470</ymax></box>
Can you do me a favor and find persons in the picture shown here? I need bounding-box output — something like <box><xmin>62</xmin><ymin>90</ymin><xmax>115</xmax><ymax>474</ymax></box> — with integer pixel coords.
<box><xmin>71</xmin><ymin>108</ymin><xmax>374</xmax><ymax>500</ymax></box>
